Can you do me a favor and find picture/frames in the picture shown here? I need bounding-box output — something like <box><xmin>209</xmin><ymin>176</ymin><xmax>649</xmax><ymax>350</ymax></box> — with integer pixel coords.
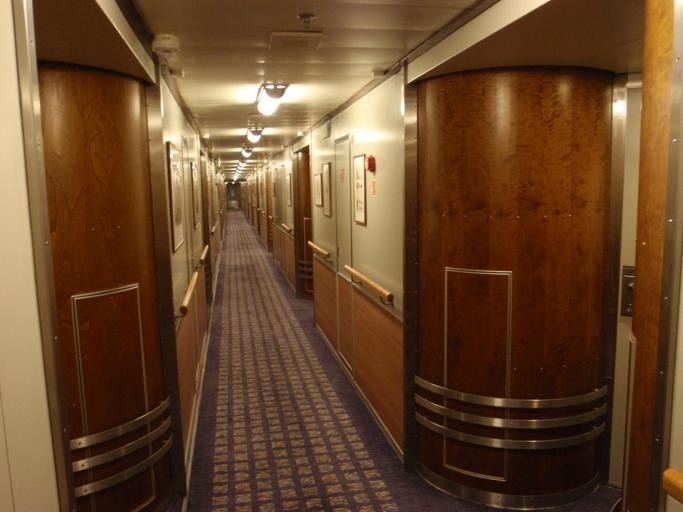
<box><xmin>191</xmin><ymin>161</ymin><xmax>201</xmax><ymax>229</ymax></box>
<box><xmin>165</xmin><ymin>141</ymin><xmax>185</xmax><ymax>252</ymax></box>
<box><xmin>285</xmin><ymin>172</ymin><xmax>290</xmax><ymax>208</ymax></box>
<box><xmin>323</xmin><ymin>164</ymin><xmax>331</xmax><ymax>216</ymax></box>
<box><xmin>313</xmin><ymin>174</ymin><xmax>322</xmax><ymax>206</ymax></box>
<box><xmin>352</xmin><ymin>154</ymin><xmax>367</xmax><ymax>225</ymax></box>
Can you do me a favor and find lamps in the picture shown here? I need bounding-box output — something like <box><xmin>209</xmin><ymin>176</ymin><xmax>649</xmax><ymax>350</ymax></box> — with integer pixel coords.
<box><xmin>238</xmin><ymin>81</ymin><xmax>288</xmax><ymax>171</ymax></box>
